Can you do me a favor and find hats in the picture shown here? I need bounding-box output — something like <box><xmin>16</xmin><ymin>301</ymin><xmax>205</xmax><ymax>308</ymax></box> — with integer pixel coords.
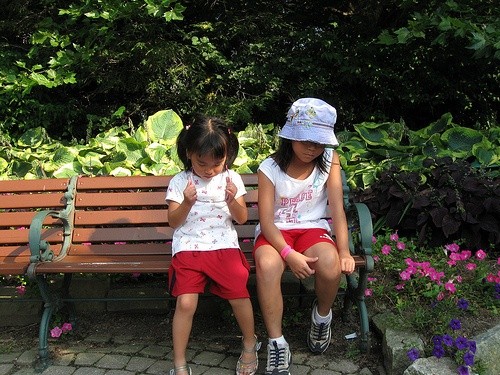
<box><xmin>277</xmin><ymin>98</ymin><xmax>339</xmax><ymax>148</ymax></box>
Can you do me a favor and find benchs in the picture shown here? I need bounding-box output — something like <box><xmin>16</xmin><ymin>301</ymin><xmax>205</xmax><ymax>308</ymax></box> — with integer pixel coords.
<box><xmin>0</xmin><ymin>170</ymin><xmax>374</xmax><ymax>371</ymax></box>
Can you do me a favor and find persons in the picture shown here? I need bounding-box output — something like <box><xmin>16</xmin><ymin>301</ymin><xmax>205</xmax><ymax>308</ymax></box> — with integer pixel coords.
<box><xmin>252</xmin><ymin>97</ymin><xmax>355</xmax><ymax>375</ymax></box>
<box><xmin>166</xmin><ymin>118</ymin><xmax>261</xmax><ymax>375</ymax></box>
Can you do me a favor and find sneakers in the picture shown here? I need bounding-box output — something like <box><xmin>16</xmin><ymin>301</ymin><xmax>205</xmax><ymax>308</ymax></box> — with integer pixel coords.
<box><xmin>266</xmin><ymin>344</ymin><xmax>292</xmax><ymax>375</ymax></box>
<box><xmin>307</xmin><ymin>308</ymin><xmax>331</xmax><ymax>353</ymax></box>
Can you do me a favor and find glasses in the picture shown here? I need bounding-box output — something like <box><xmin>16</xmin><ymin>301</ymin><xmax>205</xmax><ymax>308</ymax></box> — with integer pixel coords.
<box><xmin>291</xmin><ymin>140</ymin><xmax>309</xmax><ymax>146</ymax></box>
<box><xmin>191</xmin><ymin>165</ymin><xmax>231</xmax><ymax>203</ymax></box>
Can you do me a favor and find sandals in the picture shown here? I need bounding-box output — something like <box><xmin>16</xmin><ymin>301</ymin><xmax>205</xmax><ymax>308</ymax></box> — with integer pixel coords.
<box><xmin>170</xmin><ymin>365</ymin><xmax>192</xmax><ymax>375</ymax></box>
<box><xmin>235</xmin><ymin>335</ymin><xmax>261</xmax><ymax>375</ymax></box>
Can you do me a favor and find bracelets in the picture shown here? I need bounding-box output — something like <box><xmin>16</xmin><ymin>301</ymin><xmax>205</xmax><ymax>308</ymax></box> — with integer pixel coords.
<box><xmin>280</xmin><ymin>245</ymin><xmax>292</xmax><ymax>260</ymax></box>
